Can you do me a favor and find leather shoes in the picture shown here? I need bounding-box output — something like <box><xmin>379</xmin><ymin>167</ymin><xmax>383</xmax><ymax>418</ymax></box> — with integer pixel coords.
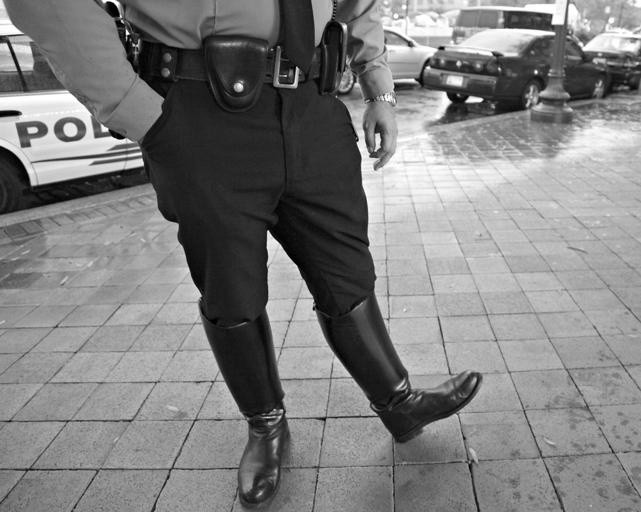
<box><xmin>309</xmin><ymin>293</ymin><xmax>484</xmax><ymax>444</ymax></box>
<box><xmin>196</xmin><ymin>297</ymin><xmax>291</xmax><ymax>508</ymax></box>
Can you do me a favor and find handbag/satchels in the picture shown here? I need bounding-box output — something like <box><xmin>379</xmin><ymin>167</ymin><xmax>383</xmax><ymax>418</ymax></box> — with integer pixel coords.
<box><xmin>320</xmin><ymin>19</ymin><xmax>347</xmax><ymax>92</ymax></box>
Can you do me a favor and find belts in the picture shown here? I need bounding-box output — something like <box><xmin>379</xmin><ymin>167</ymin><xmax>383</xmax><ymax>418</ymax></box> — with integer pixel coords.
<box><xmin>131</xmin><ymin>42</ymin><xmax>319</xmax><ymax>84</ymax></box>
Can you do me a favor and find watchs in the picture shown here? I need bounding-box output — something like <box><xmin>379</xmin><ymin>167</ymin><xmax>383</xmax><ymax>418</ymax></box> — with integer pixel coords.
<box><xmin>363</xmin><ymin>90</ymin><xmax>398</xmax><ymax>106</ymax></box>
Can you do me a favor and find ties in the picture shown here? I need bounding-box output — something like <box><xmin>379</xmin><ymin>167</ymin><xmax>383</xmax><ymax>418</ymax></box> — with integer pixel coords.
<box><xmin>277</xmin><ymin>0</ymin><xmax>315</xmax><ymax>74</ymax></box>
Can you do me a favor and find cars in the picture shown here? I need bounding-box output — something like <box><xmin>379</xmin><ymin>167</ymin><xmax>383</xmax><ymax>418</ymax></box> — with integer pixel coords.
<box><xmin>0</xmin><ymin>18</ymin><xmax>145</xmax><ymax>215</ymax></box>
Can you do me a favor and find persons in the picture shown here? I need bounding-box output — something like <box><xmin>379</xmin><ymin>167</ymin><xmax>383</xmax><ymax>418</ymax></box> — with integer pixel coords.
<box><xmin>1</xmin><ymin>0</ymin><xmax>483</xmax><ymax>511</ymax></box>
